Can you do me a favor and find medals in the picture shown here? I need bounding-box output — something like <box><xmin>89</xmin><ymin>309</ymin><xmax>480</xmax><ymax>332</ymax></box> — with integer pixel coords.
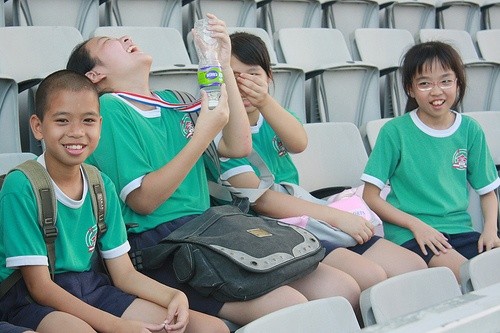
<box><xmin>234</xmin><ymin>72</ymin><xmax>242</xmax><ymax>84</ymax></box>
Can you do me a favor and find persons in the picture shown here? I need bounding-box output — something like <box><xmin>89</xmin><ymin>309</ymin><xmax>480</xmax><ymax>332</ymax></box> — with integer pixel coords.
<box><xmin>66</xmin><ymin>13</ymin><xmax>361</xmax><ymax>326</ymax></box>
<box><xmin>0</xmin><ymin>69</ymin><xmax>230</xmax><ymax>333</ymax></box>
<box><xmin>360</xmin><ymin>41</ymin><xmax>500</xmax><ymax>285</ymax></box>
<box><xmin>213</xmin><ymin>31</ymin><xmax>428</xmax><ymax>292</ymax></box>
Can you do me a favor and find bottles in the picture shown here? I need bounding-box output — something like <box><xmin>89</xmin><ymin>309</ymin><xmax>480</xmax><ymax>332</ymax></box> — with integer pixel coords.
<box><xmin>193</xmin><ymin>19</ymin><xmax>224</xmax><ymax>107</ymax></box>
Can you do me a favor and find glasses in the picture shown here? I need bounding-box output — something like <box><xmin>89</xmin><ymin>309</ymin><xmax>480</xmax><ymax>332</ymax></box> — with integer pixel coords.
<box><xmin>413</xmin><ymin>77</ymin><xmax>457</xmax><ymax>91</ymax></box>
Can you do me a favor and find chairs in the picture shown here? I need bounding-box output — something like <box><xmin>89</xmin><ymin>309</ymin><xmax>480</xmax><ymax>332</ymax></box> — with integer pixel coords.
<box><xmin>0</xmin><ymin>0</ymin><xmax>500</xmax><ymax>333</ymax></box>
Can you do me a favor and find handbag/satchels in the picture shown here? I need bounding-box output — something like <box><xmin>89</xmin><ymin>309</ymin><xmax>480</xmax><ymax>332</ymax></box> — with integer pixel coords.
<box><xmin>161</xmin><ymin>203</ymin><xmax>326</xmax><ymax>301</ymax></box>
<box><xmin>261</xmin><ymin>192</ymin><xmax>385</xmax><ymax>247</ymax></box>
